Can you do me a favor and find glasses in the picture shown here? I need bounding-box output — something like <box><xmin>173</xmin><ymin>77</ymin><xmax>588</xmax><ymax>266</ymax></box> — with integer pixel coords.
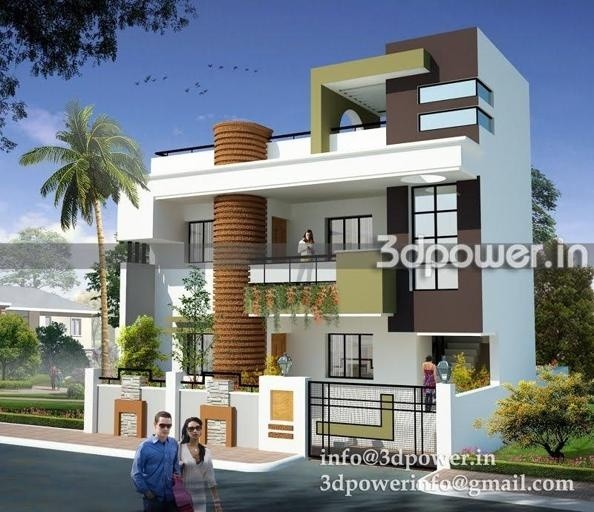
<box><xmin>158</xmin><ymin>424</ymin><xmax>171</xmax><ymax>428</ymax></box>
<box><xmin>186</xmin><ymin>425</ymin><xmax>201</xmax><ymax>432</ymax></box>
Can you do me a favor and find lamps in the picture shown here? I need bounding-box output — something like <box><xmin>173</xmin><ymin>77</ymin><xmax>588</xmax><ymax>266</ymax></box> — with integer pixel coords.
<box><xmin>436</xmin><ymin>361</ymin><xmax>451</xmax><ymax>384</ymax></box>
<box><xmin>277</xmin><ymin>354</ymin><xmax>293</xmax><ymax>376</ymax></box>
